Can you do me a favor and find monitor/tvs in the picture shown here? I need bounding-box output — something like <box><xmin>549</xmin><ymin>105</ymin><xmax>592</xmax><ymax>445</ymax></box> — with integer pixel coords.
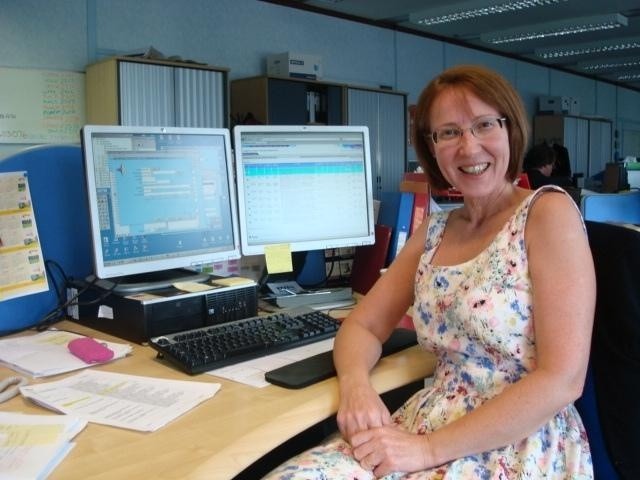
<box><xmin>79</xmin><ymin>123</ymin><xmax>242</xmax><ymax>293</ymax></box>
<box><xmin>232</xmin><ymin>124</ymin><xmax>376</xmax><ymax>313</ymax></box>
<box><xmin>605</xmin><ymin>162</ymin><xmax>624</xmax><ymax>193</ymax></box>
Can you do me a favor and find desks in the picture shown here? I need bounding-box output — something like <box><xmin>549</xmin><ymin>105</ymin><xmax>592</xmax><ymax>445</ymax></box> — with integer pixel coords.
<box><xmin>0</xmin><ymin>293</ymin><xmax>438</xmax><ymax>480</ymax></box>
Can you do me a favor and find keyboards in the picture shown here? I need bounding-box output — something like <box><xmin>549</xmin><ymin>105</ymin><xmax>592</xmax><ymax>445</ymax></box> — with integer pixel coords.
<box><xmin>149</xmin><ymin>304</ymin><xmax>343</xmax><ymax>375</ymax></box>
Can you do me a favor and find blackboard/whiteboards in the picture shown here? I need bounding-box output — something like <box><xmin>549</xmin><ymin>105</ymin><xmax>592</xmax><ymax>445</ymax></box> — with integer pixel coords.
<box><xmin>0</xmin><ymin>65</ymin><xmax>86</xmax><ymax>145</ymax></box>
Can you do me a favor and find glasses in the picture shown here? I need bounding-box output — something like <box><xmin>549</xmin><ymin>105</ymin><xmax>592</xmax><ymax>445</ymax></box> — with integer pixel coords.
<box><xmin>432</xmin><ymin>117</ymin><xmax>506</xmax><ymax>151</ymax></box>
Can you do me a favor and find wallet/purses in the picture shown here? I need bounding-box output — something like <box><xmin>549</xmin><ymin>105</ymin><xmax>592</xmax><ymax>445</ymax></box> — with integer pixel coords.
<box><xmin>68</xmin><ymin>337</ymin><xmax>114</xmax><ymax>365</ymax></box>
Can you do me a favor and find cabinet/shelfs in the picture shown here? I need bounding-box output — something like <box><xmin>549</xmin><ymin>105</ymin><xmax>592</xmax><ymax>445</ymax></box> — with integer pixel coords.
<box><xmin>533</xmin><ymin>115</ymin><xmax>589</xmax><ymax>183</ymax></box>
<box><xmin>231</xmin><ymin>74</ymin><xmax>409</xmax><ymax>197</ymax></box>
<box><xmin>589</xmin><ymin>119</ymin><xmax>614</xmax><ymax>178</ymax></box>
<box><xmin>86</xmin><ymin>58</ymin><xmax>231</xmax><ymax>131</ymax></box>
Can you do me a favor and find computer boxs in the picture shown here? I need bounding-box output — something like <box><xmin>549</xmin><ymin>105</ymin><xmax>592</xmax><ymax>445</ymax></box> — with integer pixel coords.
<box><xmin>65</xmin><ymin>269</ymin><xmax>259</xmax><ymax>344</ymax></box>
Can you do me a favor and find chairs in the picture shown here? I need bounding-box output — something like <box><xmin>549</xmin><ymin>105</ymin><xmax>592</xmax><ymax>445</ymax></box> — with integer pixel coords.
<box><xmin>580</xmin><ymin>191</ymin><xmax>640</xmax><ymax>221</ymax></box>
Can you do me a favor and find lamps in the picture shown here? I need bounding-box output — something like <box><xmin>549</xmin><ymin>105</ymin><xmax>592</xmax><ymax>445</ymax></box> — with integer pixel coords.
<box><xmin>409</xmin><ymin>0</ymin><xmax>640</xmax><ymax>81</ymax></box>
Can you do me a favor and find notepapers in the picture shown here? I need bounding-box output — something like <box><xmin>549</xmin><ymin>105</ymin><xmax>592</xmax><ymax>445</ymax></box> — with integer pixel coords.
<box><xmin>210</xmin><ymin>275</ymin><xmax>255</xmax><ymax>288</ymax></box>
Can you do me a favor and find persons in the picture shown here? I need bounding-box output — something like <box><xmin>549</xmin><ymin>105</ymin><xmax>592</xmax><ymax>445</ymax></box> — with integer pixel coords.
<box><xmin>265</xmin><ymin>63</ymin><xmax>600</xmax><ymax>480</ymax></box>
<box><xmin>525</xmin><ymin>142</ymin><xmax>572</xmax><ymax>190</ymax></box>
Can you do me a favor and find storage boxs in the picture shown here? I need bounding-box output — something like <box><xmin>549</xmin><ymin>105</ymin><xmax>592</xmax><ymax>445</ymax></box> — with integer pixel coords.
<box><xmin>538</xmin><ymin>94</ymin><xmax>581</xmax><ymax>116</ymax></box>
<box><xmin>264</xmin><ymin>50</ymin><xmax>323</xmax><ymax>81</ymax></box>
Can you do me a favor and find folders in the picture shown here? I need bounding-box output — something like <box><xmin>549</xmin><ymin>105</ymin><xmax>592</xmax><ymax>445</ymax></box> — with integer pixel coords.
<box><xmin>377</xmin><ymin>191</ymin><xmax>430</xmax><ymax>268</ymax></box>
